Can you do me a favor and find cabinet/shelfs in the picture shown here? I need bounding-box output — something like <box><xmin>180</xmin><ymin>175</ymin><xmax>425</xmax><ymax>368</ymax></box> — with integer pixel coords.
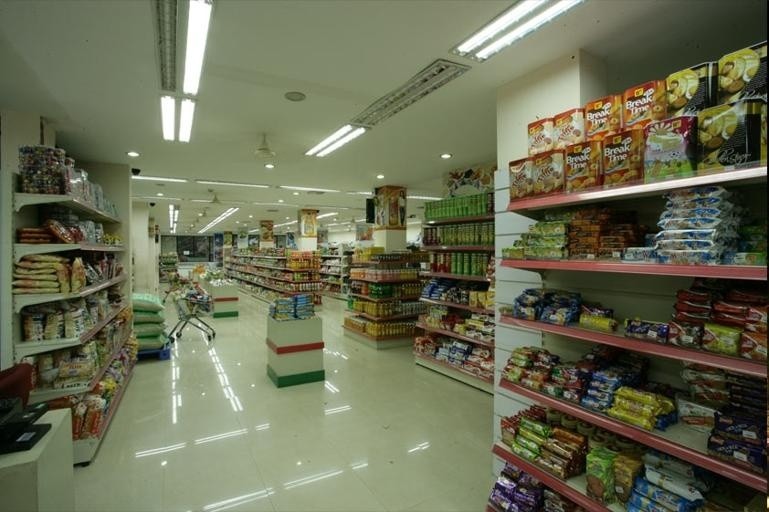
<box><xmin>319</xmin><ymin>246</ymin><xmax>353</xmax><ymax>300</ymax></box>
<box><xmin>222</xmin><ymin>247</ymin><xmax>322</xmax><ymax>310</ymax></box>
<box><xmin>344</xmin><ymin>255</ymin><xmax>422</xmax><ymax>344</ymax></box>
<box><xmin>415</xmin><ymin>203</ymin><xmax>493</xmax><ymax>378</ymax></box>
<box><xmin>14</xmin><ymin>189</ymin><xmax>134</xmax><ymax>465</ymax></box>
<box><xmin>493</xmin><ymin>167</ymin><xmax>769</xmax><ymax>512</ymax></box>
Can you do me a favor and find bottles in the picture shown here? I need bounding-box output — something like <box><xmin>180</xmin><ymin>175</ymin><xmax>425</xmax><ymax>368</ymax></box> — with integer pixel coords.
<box><xmin>291</xmin><ymin>257</ymin><xmax>320</xmax><ymax>304</ymax></box>
<box><xmin>350</xmin><ymin>254</ymin><xmax>424</xmax><ymax>336</ymax></box>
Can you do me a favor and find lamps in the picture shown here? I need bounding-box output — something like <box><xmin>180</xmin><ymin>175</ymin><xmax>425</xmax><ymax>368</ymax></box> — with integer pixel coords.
<box><xmin>197</xmin><ymin>207</ymin><xmax>239</xmax><ymax>239</ymax></box>
<box><xmin>251</xmin><ymin>210</ymin><xmax>340</xmax><ymax>236</ymax></box>
<box><xmin>252</xmin><ymin>133</ymin><xmax>276</xmax><ymax>158</ymax></box>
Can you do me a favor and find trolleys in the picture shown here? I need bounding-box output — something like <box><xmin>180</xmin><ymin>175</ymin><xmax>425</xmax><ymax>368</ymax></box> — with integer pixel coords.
<box><xmin>161</xmin><ymin>268</ymin><xmax>216</xmax><ymax>343</ymax></box>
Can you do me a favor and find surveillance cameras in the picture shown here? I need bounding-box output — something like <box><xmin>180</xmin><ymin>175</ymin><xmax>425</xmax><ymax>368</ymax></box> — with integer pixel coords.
<box><xmin>149</xmin><ymin>203</ymin><xmax>155</xmax><ymax>206</ymax></box>
<box><xmin>132</xmin><ymin>169</ymin><xmax>140</xmax><ymax>175</ymax></box>
